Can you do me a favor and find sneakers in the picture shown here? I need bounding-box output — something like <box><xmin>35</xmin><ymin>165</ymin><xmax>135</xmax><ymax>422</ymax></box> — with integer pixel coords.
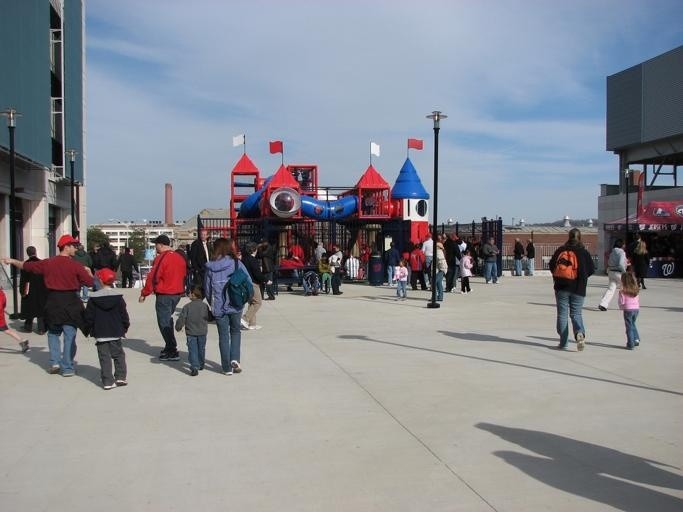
<box><xmin>231</xmin><ymin>360</ymin><xmax>241</xmax><ymax>373</ymax></box>
<box><xmin>160</xmin><ymin>351</ymin><xmax>180</xmax><ymax>360</ymax></box>
<box><xmin>577</xmin><ymin>333</ymin><xmax>585</xmax><ymax>351</ymax></box>
<box><xmin>241</xmin><ymin>318</ymin><xmax>248</xmax><ymax>328</ymax></box>
<box><xmin>104</xmin><ymin>382</ymin><xmax>116</xmax><ymax>388</ymax></box>
<box><xmin>19</xmin><ymin>339</ymin><xmax>28</xmax><ymax>353</ymax></box>
<box><xmin>192</xmin><ymin>369</ymin><xmax>197</xmax><ymax>375</ymax></box>
<box><xmin>248</xmin><ymin>325</ymin><xmax>261</xmax><ymax>330</ymax></box>
<box><xmin>627</xmin><ymin>343</ymin><xmax>634</xmax><ymax>350</ymax></box>
<box><xmin>635</xmin><ymin>340</ymin><xmax>639</xmax><ymax>346</ymax></box>
<box><xmin>117</xmin><ymin>380</ymin><xmax>127</xmax><ymax>386</ymax></box>
<box><xmin>63</xmin><ymin>372</ymin><xmax>74</xmax><ymax>377</ymax></box>
<box><xmin>48</xmin><ymin>364</ymin><xmax>59</xmax><ymax>374</ymax></box>
<box><xmin>599</xmin><ymin>305</ymin><xmax>606</xmax><ymax>311</ymax></box>
<box><xmin>161</xmin><ymin>349</ymin><xmax>177</xmax><ymax>355</ymax></box>
<box><xmin>559</xmin><ymin>344</ymin><xmax>568</xmax><ymax>351</ymax></box>
<box><xmin>222</xmin><ymin>371</ymin><xmax>233</xmax><ymax>375</ymax></box>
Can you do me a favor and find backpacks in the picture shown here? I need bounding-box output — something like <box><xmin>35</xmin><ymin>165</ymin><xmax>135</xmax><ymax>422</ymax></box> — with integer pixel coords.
<box><xmin>553</xmin><ymin>247</ymin><xmax>578</xmax><ymax>280</ymax></box>
<box><xmin>223</xmin><ymin>259</ymin><xmax>253</xmax><ymax>311</ymax></box>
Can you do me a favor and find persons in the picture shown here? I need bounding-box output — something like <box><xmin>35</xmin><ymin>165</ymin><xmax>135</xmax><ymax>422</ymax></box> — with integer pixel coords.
<box><xmin>549</xmin><ymin>230</ymin><xmax>676</xmax><ymax>352</ymax></box>
<box><xmin>140</xmin><ymin>230</ymin><xmax>273</xmax><ymax>376</ymax></box>
<box><xmin>514</xmin><ymin>238</ymin><xmax>534</xmax><ymax>276</ymax></box>
<box><xmin>280</xmin><ymin>239</ymin><xmax>343</xmax><ymax>295</ymax></box>
<box><xmin>385</xmin><ymin>231</ymin><xmax>500</xmax><ymax>301</ymax></box>
<box><xmin>2</xmin><ymin>237</ymin><xmax>139</xmax><ymax>389</ymax></box>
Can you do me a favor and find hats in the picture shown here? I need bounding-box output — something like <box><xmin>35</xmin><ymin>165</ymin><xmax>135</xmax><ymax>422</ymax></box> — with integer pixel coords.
<box><xmin>150</xmin><ymin>235</ymin><xmax>170</xmax><ymax>245</ymax></box>
<box><xmin>57</xmin><ymin>236</ymin><xmax>79</xmax><ymax>247</ymax></box>
<box><xmin>97</xmin><ymin>269</ymin><xmax>115</xmax><ymax>285</ymax></box>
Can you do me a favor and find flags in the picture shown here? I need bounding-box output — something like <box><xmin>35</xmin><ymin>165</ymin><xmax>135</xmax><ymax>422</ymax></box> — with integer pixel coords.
<box><xmin>269</xmin><ymin>141</ymin><xmax>282</xmax><ymax>154</ymax></box>
<box><xmin>233</xmin><ymin>134</ymin><xmax>244</xmax><ymax>146</ymax></box>
<box><xmin>371</xmin><ymin>142</ymin><xmax>380</xmax><ymax>157</ymax></box>
<box><xmin>407</xmin><ymin>138</ymin><xmax>423</xmax><ymax>150</ymax></box>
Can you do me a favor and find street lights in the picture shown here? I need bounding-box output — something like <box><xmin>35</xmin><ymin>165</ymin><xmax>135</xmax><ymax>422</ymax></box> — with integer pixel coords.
<box><xmin>66</xmin><ymin>149</ymin><xmax>79</xmax><ymax>245</ymax></box>
<box><xmin>0</xmin><ymin>107</ymin><xmax>26</xmax><ymax>320</ymax></box>
<box><xmin>425</xmin><ymin>110</ymin><xmax>448</xmax><ymax>308</ymax></box>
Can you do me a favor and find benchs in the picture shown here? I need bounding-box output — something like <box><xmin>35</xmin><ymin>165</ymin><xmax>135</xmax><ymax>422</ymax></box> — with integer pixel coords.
<box><xmin>274</xmin><ymin>268</ymin><xmax>340</xmax><ymax>294</ymax></box>
